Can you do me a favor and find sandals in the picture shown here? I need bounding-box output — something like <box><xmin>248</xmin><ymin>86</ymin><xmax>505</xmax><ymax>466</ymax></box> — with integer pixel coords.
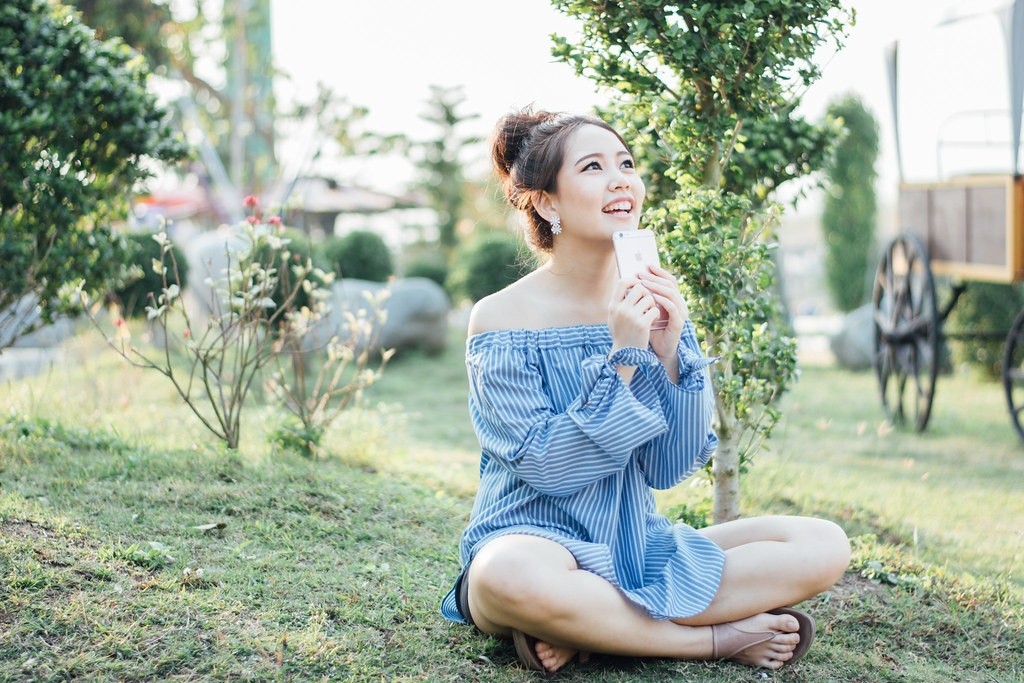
<box><xmin>512</xmin><ymin>627</ymin><xmax>590</xmax><ymax>672</ymax></box>
<box><xmin>711</xmin><ymin>606</ymin><xmax>815</xmax><ymax>671</ymax></box>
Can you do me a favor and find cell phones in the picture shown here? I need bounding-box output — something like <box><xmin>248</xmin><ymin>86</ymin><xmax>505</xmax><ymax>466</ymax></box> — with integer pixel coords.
<box><xmin>612</xmin><ymin>229</ymin><xmax>670</xmax><ymax>331</ymax></box>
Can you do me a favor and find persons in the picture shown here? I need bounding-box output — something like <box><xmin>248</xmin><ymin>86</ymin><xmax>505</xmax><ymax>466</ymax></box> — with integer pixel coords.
<box><xmin>441</xmin><ymin>102</ymin><xmax>851</xmax><ymax>675</ymax></box>
